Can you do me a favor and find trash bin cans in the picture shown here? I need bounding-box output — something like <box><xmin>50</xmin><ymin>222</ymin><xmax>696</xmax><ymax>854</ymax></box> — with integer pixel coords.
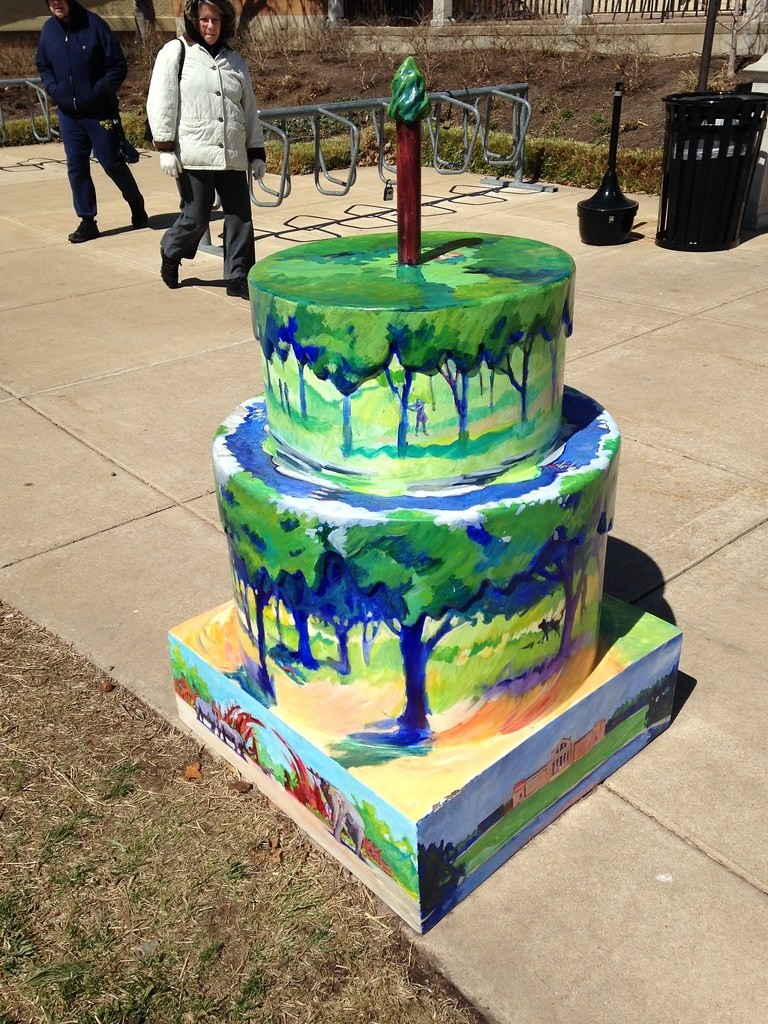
<box><xmin>652</xmin><ymin>91</ymin><xmax>768</xmax><ymax>252</ymax></box>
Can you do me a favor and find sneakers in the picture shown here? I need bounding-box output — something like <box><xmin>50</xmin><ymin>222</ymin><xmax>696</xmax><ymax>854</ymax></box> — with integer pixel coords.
<box><xmin>68</xmin><ymin>220</ymin><xmax>100</xmax><ymax>242</ymax></box>
<box><xmin>226</xmin><ymin>277</ymin><xmax>250</xmax><ymax>300</ymax></box>
<box><xmin>160</xmin><ymin>247</ymin><xmax>182</xmax><ymax>289</ymax></box>
<box><xmin>131</xmin><ymin>195</ymin><xmax>148</xmax><ymax>229</ymax></box>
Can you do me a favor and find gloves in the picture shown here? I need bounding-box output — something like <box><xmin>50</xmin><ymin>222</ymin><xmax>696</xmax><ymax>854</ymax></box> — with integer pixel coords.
<box><xmin>253</xmin><ymin>159</ymin><xmax>265</xmax><ymax>180</ymax></box>
<box><xmin>160</xmin><ymin>151</ymin><xmax>182</xmax><ymax>178</ymax></box>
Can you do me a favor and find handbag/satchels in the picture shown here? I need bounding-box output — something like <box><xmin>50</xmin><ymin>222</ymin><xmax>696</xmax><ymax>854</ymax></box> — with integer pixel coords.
<box><xmin>144</xmin><ymin>38</ymin><xmax>185</xmax><ymax>143</ymax></box>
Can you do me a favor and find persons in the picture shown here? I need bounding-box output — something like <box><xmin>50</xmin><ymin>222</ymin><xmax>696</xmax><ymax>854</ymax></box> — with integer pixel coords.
<box><xmin>35</xmin><ymin>0</ymin><xmax>150</xmax><ymax>245</ymax></box>
<box><xmin>145</xmin><ymin>0</ymin><xmax>266</xmax><ymax>302</ymax></box>
